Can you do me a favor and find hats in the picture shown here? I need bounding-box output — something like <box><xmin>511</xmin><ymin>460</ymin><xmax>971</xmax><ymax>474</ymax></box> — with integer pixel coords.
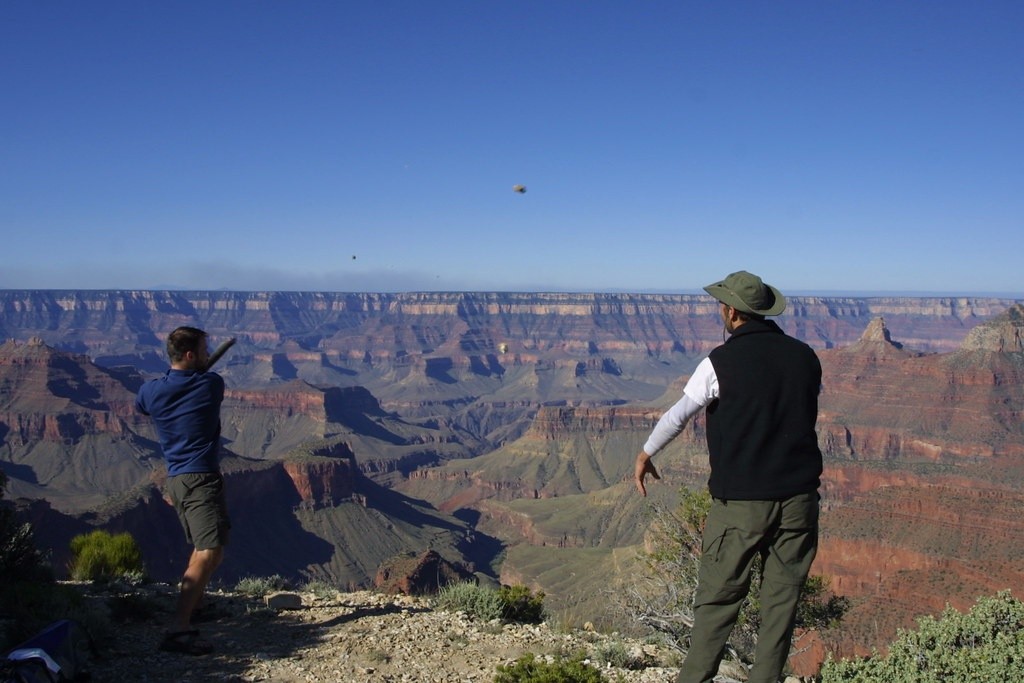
<box><xmin>704</xmin><ymin>270</ymin><xmax>787</xmax><ymax>315</ymax></box>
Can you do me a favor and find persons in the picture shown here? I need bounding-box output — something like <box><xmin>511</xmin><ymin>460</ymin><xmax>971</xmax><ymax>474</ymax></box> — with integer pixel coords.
<box><xmin>633</xmin><ymin>270</ymin><xmax>824</xmax><ymax>682</ymax></box>
<box><xmin>132</xmin><ymin>326</ymin><xmax>226</xmax><ymax>656</ymax></box>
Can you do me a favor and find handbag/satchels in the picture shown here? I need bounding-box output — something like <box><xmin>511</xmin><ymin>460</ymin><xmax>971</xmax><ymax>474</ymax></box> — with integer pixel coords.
<box><xmin>0</xmin><ymin>618</ymin><xmax>99</xmax><ymax>683</ymax></box>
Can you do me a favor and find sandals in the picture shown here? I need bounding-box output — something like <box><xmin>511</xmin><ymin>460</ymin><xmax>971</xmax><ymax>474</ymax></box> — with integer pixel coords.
<box><xmin>159</xmin><ymin>628</ymin><xmax>213</xmax><ymax>655</ymax></box>
<box><xmin>189</xmin><ymin>603</ymin><xmax>232</xmax><ymax>623</ymax></box>
